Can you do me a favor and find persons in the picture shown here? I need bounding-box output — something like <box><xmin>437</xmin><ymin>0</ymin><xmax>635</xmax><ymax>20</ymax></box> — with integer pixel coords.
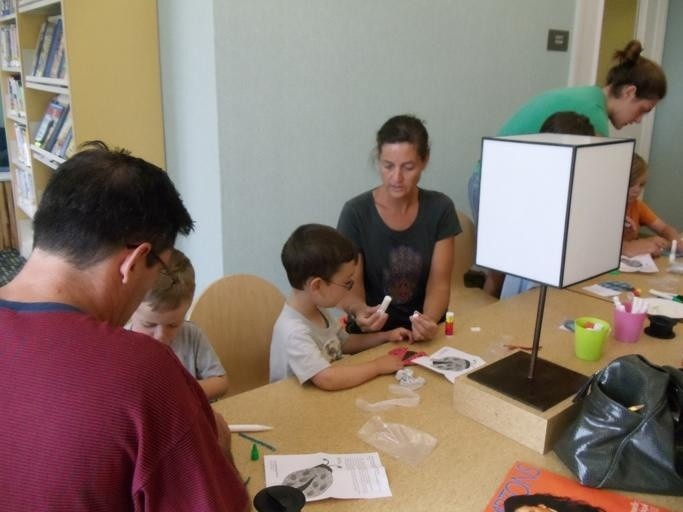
<box><xmin>0</xmin><ymin>140</ymin><xmax>251</xmax><ymax>511</ymax></box>
<box><xmin>500</xmin><ymin>111</ymin><xmax>595</xmax><ymax>302</ymax></box>
<box><xmin>504</xmin><ymin>493</ymin><xmax>607</xmax><ymax>512</ymax></box>
<box><xmin>621</xmin><ymin>153</ymin><xmax>683</xmax><ymax>254</ymax></box>
<box><xmin>123</xmin><ymin>248</ymin><xmax>230</xmax><ymax>401</ymax></box>
<box><xmin>467</xmin><ymin>41</ymin><xmax>667</xmax><ymax>302</ymax></box>
<box><xmin>337</xmin><ymin>115</ymin><xmax>463</xmax><ymax>342</ymax></box>
<box><xmin>269</xmin><ymin>225</ymin><xmax>415</xmax><ymax>391</ymax></box>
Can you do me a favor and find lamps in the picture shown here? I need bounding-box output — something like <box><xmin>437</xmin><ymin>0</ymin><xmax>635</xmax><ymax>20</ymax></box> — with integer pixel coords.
<box><xmin>451</xmin><ymin>132</ymin><xmax>638</xmax><ymax>455</ymax></box>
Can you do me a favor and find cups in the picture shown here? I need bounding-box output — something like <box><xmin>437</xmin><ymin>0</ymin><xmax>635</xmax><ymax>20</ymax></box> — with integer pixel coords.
<box><xmin>573</xmin><ymin>317</ymin><xmax>610</xmax><ymax>362</ymax></box>
<box><xmin>613</xmin><ymin>303</ymin><xmax>648</xmax><ymax>343</ymax></box>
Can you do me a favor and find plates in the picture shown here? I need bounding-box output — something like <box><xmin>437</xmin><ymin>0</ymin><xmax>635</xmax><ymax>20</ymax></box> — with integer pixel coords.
<box><xmin>646</xmin><ymin>299</ymin><xmax>683</xmax><ymax>339</ymax></box>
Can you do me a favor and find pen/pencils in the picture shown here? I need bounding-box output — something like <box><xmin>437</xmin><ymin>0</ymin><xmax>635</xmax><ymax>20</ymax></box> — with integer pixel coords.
<box><xmin>238</xmin><ymin>432</ymin><xmax>276</xmax><ymax>452</ymax></box>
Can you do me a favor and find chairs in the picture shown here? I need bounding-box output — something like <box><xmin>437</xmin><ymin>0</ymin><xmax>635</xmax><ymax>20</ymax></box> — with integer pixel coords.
<box><xmin>446</xmin><ymin>212</ymin><xmax>500</xmax><ymax>317</ymax></box>
<box><xmin>187</xmin><ymin>273</ymin><xmax>287</xmax><ymax>406</ymax></box>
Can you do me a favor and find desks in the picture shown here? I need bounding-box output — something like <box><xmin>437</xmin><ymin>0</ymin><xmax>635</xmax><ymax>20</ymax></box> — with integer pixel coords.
<box><xmin>565</xmin><ymin>241</ymin><xmax>682</xmax><ymax>303</ymax></box>
<box><xmin>208</xmin><ymin>245</ymin><xmax>683</xmax><ymax>512</ymax></box>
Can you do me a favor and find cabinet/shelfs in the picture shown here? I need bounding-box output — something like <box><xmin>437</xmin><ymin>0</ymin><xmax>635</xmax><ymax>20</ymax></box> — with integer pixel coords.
<box><xmin>1</xmin><ymin>0</ymin><xmax>167</xmax><ymax>269</ymax></box>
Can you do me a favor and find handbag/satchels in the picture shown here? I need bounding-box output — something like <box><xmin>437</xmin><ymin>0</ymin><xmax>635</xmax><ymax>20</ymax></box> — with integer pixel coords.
<box><xmin>548</xmin><ymin>353</ymin><xmax>683</xmax><ymax>498</ymax></box>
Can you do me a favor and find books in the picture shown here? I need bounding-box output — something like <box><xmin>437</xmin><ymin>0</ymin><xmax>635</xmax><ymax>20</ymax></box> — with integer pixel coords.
<box><xmin>9</xmin><ymin>122</ymin><xmax>36</xmax><ymax>206</ymax></box>
<box><xmin>34</xmin><ymin>95</ymin><xmax>75</xmax><ymax>160</ymax></box>
<box><xmin>1</xmin><ymin>22</ymin><xmax>23</xmax><ymax>68</ymax></box>
<box><xmin>484</xmin><ymin>461</ymin><xmax>672</xmax><ymax>512</ymax></box>
<box><xmin>3</xmin><ymin>76</ymin><xmax>25</xmax><ymax>119</ymax></box>
<box><xmin>31</xmin><ymin>13</ymin><xmax>67</xmax><ymax>79</ymax></box>
<box><xmin>1</xmin><ymin>1</ymin><xmax>15</xmax><ymax>18</ymax></box>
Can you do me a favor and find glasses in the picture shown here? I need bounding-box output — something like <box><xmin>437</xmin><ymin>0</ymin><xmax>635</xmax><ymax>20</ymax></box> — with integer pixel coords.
<box><xmin>326</xmin><ymin>278</ymin><xmax>356</xmax><ymax>291</ymax></box>
<box><xmin>124</xmin><ymin>243</ymin><xmax>181</xmax><ymax>301</ymax></box>
